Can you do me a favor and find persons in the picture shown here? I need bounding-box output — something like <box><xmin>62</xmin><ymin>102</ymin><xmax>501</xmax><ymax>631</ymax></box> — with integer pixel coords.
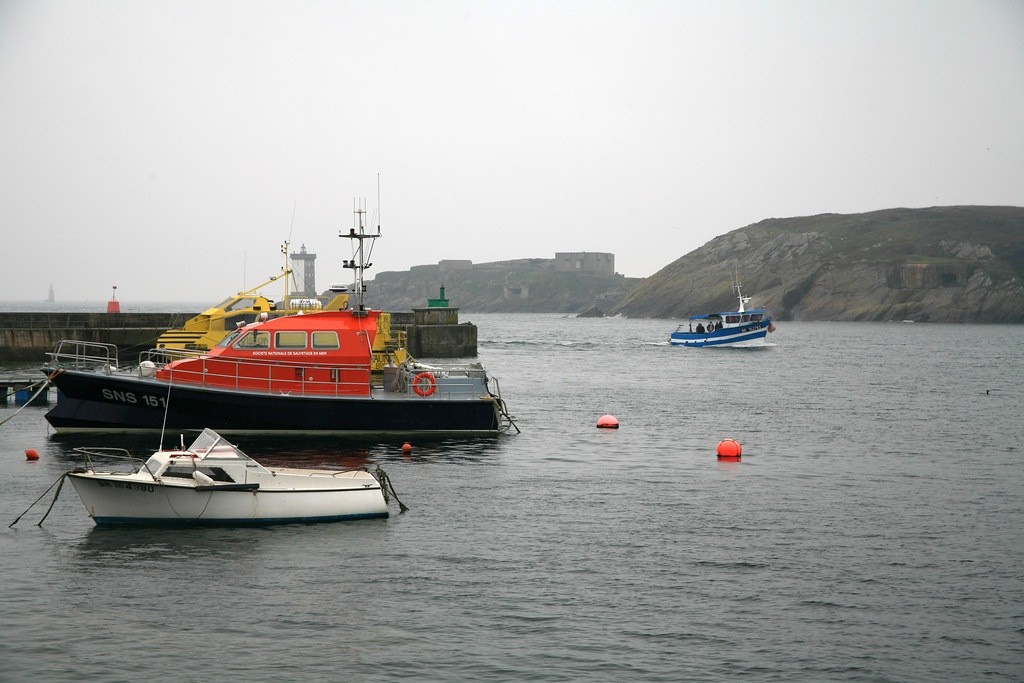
<box><xmin>715</xmin><ymin>321</ymin><xmax>723</xmax><ymax>330</ymax></box>
<box><xmin>707</xmin><ymin>321</ymin><xmax>714</xmax><ymax>333</ymax></box>
<box><xmin>696</xmin><ymin>323</ymin><xmax>705</xmax><ymax>334</ymax></box>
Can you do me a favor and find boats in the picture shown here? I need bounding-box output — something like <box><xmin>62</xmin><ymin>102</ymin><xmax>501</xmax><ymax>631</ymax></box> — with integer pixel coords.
<box><xmin>41</xmin><ymin>174</ymin><xmax>505</xmax><ymax>444</ymax></box>
<box><xmin>155</xmin><ymin>240</ymin><xmax>410</xmax><ymax>373</ymax></box>
<box><xmin>65</xmin><ymin>428</ymin><xmax>391</xmax><ymax>529</ymax></box>
<box><xmin>666</xmin><ymin>266</ymin><xmax>772</xmax><ymax>347</ymax></box>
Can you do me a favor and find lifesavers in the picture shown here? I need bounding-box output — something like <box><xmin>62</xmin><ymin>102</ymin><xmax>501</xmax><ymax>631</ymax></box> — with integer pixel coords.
<box><xmin>413</xmin><ymin>373</ymin><xmax>436</xmax><ymax>397</ymax></box>
